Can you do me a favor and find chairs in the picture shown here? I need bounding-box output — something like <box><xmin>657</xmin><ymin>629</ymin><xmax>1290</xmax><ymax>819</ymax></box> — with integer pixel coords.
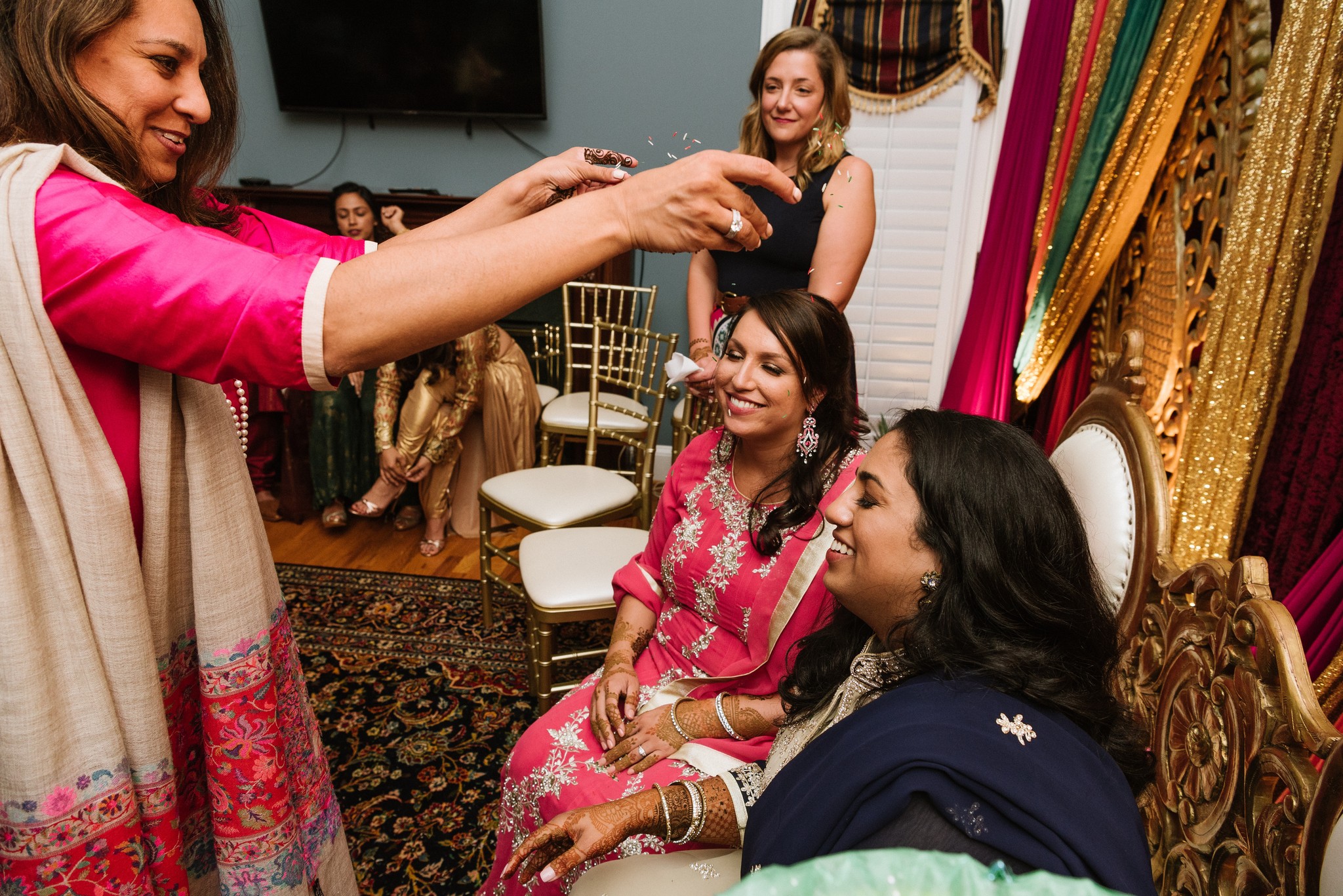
<box><xmin>1048</xmin><ymin>328</ymin><xmax>1171</xmax><ymax>650</ymax></box>
<box><xmin>518</xmin><ymin>384</ymin><xmax>723</xmax><ymax>713</ymax></box>
<box><xmin>478</xmin><ymin>316</ymin><xmax>680</xmax><ymax>632</ymax></box>
<box><xmin>540</xmin><ymin>281</ymin><xmax>658</xmax><ymax>490</ymax></box>
<box><xmin>531</xmin><ymin>324</ymin><xmax>561</xmax><ymax>407</ymax></box>
<box><xmin>1109</xmin><ymin>556</ymin><xmax>1343</xmax><ymax>896</ymax></box>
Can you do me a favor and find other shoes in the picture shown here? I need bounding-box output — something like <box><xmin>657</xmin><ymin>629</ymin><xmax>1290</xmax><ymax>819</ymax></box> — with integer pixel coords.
<box><xmin>395</xmin><ymin>506</ymin><xmax>419</xmax><ymax>531</ymax></box>
<box><xmin>256</xmin><ymin>494</ymin><xmax>283</xmax><ymax>520</ymax></box>
<box><xmin>323</xmin><ymin>505</ymin><xmax>345</xmax><ymax>527</ymax></box>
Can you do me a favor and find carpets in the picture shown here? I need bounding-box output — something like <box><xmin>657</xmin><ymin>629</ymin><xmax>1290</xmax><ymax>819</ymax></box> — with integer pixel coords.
<box><xmin>271</xmin><ymin>562</ymin><xmax>614</xmax><ymax>895</ymax></box>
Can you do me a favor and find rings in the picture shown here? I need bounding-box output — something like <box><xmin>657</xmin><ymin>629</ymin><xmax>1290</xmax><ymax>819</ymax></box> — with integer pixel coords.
<box><xmin>638</xmin><ymin>746</ymin><xmax>646</xmax><ymax>756</ymax></box>
<box><xmin>724</xmin><ymin>208</ymin><xmax>743</xmax><ymax>239</ymax></box>
<box><xmin>709</xmin><ymin>388</ymin><xmax>713</xmax><ymax>393</ymax></box>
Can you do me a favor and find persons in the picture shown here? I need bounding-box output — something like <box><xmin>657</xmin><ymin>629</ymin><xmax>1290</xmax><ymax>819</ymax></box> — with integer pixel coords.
<box><xmin>501</xmin><ymin>410</ymin><xmax>1152</xmax><ymax>896</ymax></box>
<box><xmin>0</xmin><ymin>0</ymin><xmax>802</xmax><ymax>896</ymax></box>
<box><xmin>248</xmin><ymin>181</ymin><xmax>540</xmax><ymax>557</ymax></box>
<box><xmin>674</xmin><ymin>25</ymin><xmax>876</xmax><ymax>457</ymax></box>
<box><xmin>481</xmin><ymin>290</ymin><xmax>872</xmax><ymax>896</ymax></box>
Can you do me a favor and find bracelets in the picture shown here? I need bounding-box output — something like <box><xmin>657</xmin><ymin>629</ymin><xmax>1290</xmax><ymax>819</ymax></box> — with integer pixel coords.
<box><xmin>671</xmin><ymin>697</ymin><xmax>698</xmax><ymax>741</ymax></box>
<box><xmin>715</xmin><ymin>691</ymin><xmax>747</xmax><ymax>741</ymax></box>
<box><xmin>651</xmin><ymin>779</ymin><xmax>707</xmax><ymax>845</ymax></box>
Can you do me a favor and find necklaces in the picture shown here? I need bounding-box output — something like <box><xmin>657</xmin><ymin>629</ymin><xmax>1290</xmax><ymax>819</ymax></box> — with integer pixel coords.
<box><xmin>782</xmin><ymin>164</ymin><xmax>798</xmax><ymax>173</ymax></box>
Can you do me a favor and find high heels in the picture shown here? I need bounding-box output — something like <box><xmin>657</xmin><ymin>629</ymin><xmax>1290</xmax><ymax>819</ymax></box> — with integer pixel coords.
<box><xmin>420</xmin><ymin>507</ymin><xmax>451</xmax><ymax>556</ymax></box>
<box><xmin>348</xmin><ymin>478</ymin><xmax>405</xmax><ymax>517</ymax></box>
